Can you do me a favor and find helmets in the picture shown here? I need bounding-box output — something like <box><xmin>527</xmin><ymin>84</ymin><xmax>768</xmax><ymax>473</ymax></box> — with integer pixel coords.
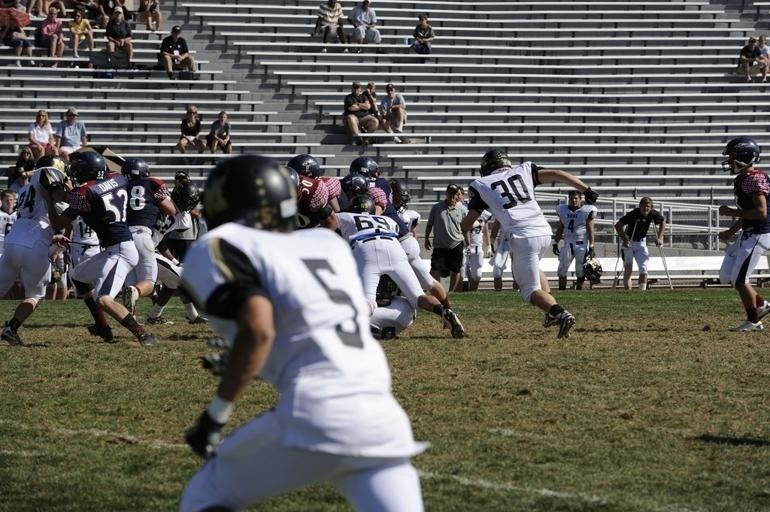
<box><xmin>720</xmin><ymin>136</ymin><xmax>761</xmax><ymax>176</ymax></box>
<box><xmin>69</xmin><ymin>152</ymin><xmax>109</xmax><ymax>188</ymax></box>
<box><xmin>387</xmin><ymin>178</ymin><xmax>410</xmax><ymax>215</ymax></box>
<box><xmin>205</xmin><ymin>156</ymin><xmax>299</xmax><ymax>233</ymax></box>
<box><xmin>341</xmin><ymin>157</ymin><xmax>381</xmax><ymax>215</ymax></box>
<box><xmin>121</xmin><ymin>157</ymin><xmax>152</xmax><ymax>183</ymax></box>
<box><xmin>584</xmin><ymin>258</ymin><xmax>602</xmax><ymax>285</ymax></box>
<box><xmin>480</xmin><ymin>149</ymin><xmax>512</xmax><ymax>177</ymax></box>
<box><xmin>171</xmin><ymin>181</ymin><xmax>202</xmax><ymax>214</ymax></box>
<box><xmin>35</xmin><ymin>154</ymin><xmax>66</xmax><ymax>173</ymax></box>
<box><xmin>288</xmin><ymin>154</ymin><xmax>321</xmax><ymax>179</ymax></box>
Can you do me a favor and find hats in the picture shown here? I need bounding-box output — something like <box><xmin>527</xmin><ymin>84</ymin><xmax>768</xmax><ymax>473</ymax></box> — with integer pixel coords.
<box><xmin>387</xmin><ymin>85</ymin><xmax>395</xmax><ymax>92</ymax></box>
<box><xmin>447</xmin><ymin>184</ymin><xmax>460</xmax><ymax>195</ymax></box>
<box><xmin>171</xmin><ymin>25</ymin><xmax>182</xmax><ymax>34</ymax></box>
<box><xmin>114</xmin><ymin>6</ymin><xmax>124</xmax><ymax>16</ymax></box>
<box><xmin>352</xmin><ymin>81</ymin><xmax>361</xmax><ymax>89</ymax></box>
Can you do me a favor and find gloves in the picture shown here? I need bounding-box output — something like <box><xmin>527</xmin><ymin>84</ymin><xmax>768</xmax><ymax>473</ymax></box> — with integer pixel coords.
<box><xmin>583</xmin><ymin>187</ymin><xmax>600</xmax><ymax>204</ymax></box>
<box><xmin>552</xmin><ymin>243</ymin><xmax>560</xmax><ymax>256</ymax></box>
<box><xmin>184</xmin><ymin>411</ymin><xmax>226</xmax><ymax>460</ymax></box>
<box><xmin>587</xmin><ymin>247</ymin><xmax>596</xmax><ymax>260</ymax></box>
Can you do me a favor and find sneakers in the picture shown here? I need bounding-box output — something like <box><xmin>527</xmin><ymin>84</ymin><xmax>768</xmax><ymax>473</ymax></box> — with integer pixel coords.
<box><xmin>392</xmin><ymin>127</ymin><xmax>403</xmax><ymax>142</ymax></box>
<box><xmin>185</xmin><ymin>315</ymin><xmax>208</xmax><ymax>324</ymax></box>
<box><xmin>734</xmin><ymin>320</ymin><xmax>764</xmax><ymax>333</ymax></box>
<box><xmin>139</xmin><ymin>332</ymin><xmax>160</xmax><ymax>348</ymax></box>
<box><xmin>441</xmin><ymin>308</ymin><xmax>463</xmax><ymax>339</ymax></box>
<box><xmin>145</xmin><ymin>317</ymin><xmax>175</xmax><ymax>327</ymax></box>
<box><xmin>122</xmin><ymin>286</ymin><xmax>138</xmax><ymax>321</ymax></box>
<box><xmin>757</xmin><ymin>299</ymin><xmax>770</xmax><ymax>318</ymax></box>
<box><xmin>555</xmin><ymin>311</ymin><xmax>574</xmax><ymax>339</ymax></box>
<box><xmin>542</xmin><ymin>311</ymin><xmax>561</xmax><ymax>327</ymax></box>
<box><xmin>88</xmin><ymin>324</ymin><xmax>113</xmax><ymax>343</ymax></box>
<box><xmin>0</xmin><ymin>325</ymin><xmax>25</xmax><ymax>347</ymax></box>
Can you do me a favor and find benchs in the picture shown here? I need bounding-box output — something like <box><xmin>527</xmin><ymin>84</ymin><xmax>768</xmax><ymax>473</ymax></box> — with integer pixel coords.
<box><xmin>0</xmin><ymin>0</ymin><xmax>770</xmax><ymax>223</ymax></box>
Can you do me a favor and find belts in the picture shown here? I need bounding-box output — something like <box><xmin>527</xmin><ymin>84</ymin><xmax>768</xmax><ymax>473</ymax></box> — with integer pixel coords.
<box><xmin>351</xmin><ymin>236</ymin><xmax>392</xmax><ymax>249</ymax></box>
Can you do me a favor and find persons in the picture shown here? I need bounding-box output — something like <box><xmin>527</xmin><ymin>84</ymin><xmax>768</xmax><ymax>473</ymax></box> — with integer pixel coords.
<box><xmin>181</xmin><ymin>153</ymin><xmax>432</xmax><ymax>512</ymax></box>
<box><xmin>1</xmin><ymin>1</ymin><xmax>465</xmax><ymax>347</ymax></box>
<box><xmin>740</xmin><ymin>35</ymin><xmax>769</xmax><ymax>83</ymax></box>
<box><xmin>455</xmin><ymin>136</ymin><xmax>770</xmax><ymax>337</ymax></box>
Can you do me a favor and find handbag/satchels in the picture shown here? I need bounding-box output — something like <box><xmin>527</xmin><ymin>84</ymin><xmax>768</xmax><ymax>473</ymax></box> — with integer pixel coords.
<box><xmin>33</xmin><ymin>29</ymin><xmax>50</xmax><ymax>47</ymax></box>
